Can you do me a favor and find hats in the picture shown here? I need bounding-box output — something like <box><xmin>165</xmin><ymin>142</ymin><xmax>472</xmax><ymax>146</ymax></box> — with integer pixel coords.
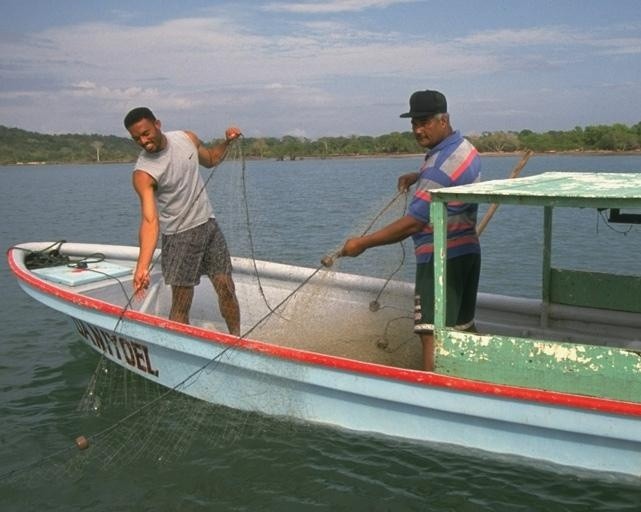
<box><xmin>400</xmin><ymin>90</ymin><xmax>447</xmax><ymax>117</ymax></box>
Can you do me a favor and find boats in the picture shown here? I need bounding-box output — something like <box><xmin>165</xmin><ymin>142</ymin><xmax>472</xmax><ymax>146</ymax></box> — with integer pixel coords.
<box><xmin>8</xmin><ymin>171</ymin><xmax>641</xmax><ymax>476</ymax></box>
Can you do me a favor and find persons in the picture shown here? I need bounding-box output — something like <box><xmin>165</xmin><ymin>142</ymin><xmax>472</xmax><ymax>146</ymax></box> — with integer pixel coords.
<box><xmin>125</xmin><ymin>107</ymin><xmax>240</xmax><ymax>338</ymax></box>
<box><xmin>343</xmin><ymin>90</ymin><xmax>481</xmax><ymax>371</ymax></box>
